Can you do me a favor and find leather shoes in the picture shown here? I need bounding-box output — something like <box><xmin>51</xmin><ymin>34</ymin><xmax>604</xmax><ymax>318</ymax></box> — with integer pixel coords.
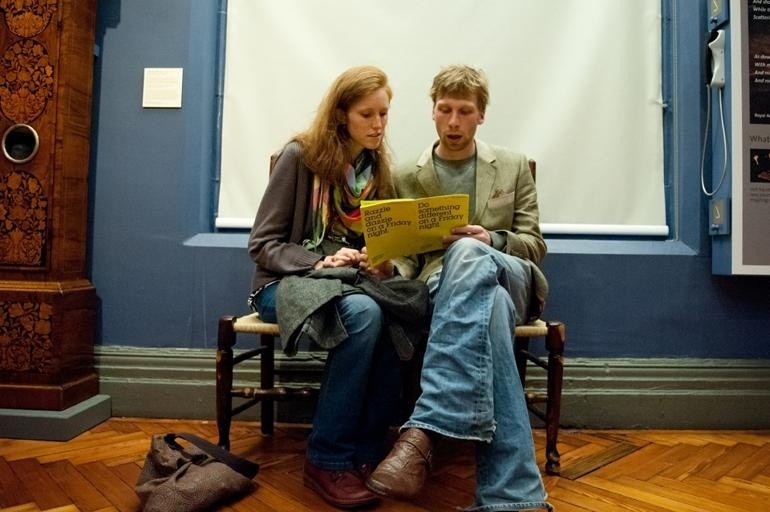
<box><xmin>363</xmin><ymin>428</ymin><xmax>434</xmax><ymax>501</ymax></box>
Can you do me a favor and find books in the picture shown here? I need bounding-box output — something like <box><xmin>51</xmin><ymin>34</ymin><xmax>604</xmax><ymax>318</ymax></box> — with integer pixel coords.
<box><xmin>358</xmin><ymin>194</ymin><xmax>472</xmax><ymax>268</ymax></box>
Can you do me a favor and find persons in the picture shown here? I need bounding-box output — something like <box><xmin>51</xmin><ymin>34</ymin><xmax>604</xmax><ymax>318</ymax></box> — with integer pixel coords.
<box><xmin>357</xmin><ymin>66</ymin><xmax>556</xmax><ymax>511</ymax></box>
<box><xmin>246</xmin><ymin>64</ymin><xmax>439</xmax><ymax>511</ymax></box>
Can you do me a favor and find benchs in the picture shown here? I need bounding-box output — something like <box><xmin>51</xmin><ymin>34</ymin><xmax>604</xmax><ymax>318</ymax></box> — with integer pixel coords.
<box><xmin>211</xmin><ymin>153</ymin><xmax>570</xmax><ymax>481</ymax></box>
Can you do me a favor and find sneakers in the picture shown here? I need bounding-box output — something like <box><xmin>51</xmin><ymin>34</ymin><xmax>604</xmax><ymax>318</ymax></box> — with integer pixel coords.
<box><xmin>300</xmin><ymin>456</ymin><xmax>380</xmax><ymax>509</ymax></box>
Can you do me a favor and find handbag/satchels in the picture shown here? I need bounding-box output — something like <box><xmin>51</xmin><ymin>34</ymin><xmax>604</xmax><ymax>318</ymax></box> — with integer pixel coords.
<box><xmin>133</xmin><ymin>431</ymin><xmax>260</xmax><ymax>512</ymax></box>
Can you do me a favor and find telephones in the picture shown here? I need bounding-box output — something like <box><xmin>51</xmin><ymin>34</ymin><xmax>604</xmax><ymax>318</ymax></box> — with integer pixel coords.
<box><xmin>705</xmin><ymin>29</ymin><xmax>725</xmax><ymax>87</ymax></box>
